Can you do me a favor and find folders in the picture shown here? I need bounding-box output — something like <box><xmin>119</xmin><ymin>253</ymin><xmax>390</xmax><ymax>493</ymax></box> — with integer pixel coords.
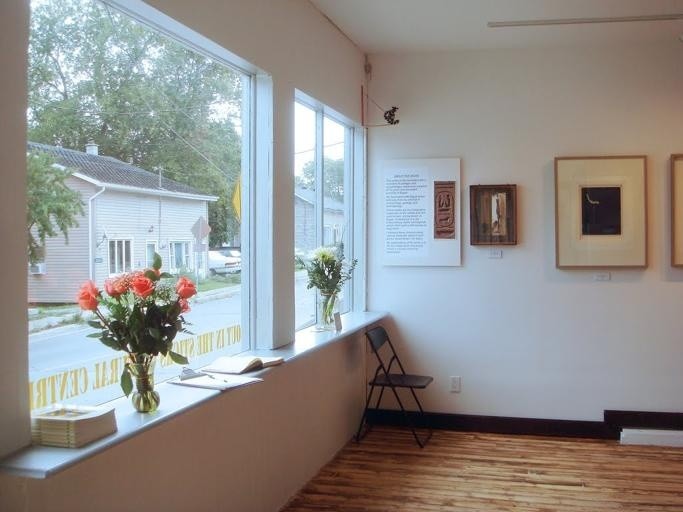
<box><xmin>166</xmin><ymin>366</ymin><xmax>264</xmax><ymax>392</ymax></box>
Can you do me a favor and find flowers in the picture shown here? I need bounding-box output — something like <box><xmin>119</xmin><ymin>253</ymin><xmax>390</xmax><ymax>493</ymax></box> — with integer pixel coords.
<box><xmin>74</xmin><ymin>251</ymin><xmax>197</xmax><ymax>398</ymax></box>
<box><xmin>295</xmin><ymin>242</ymin><xmax>359</xmax><ymax>324</ymax></box>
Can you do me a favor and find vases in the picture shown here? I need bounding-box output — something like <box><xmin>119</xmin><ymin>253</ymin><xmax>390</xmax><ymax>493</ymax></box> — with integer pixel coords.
<box><xmin>126</xmin><ymin>362</ymin><xmax>160</xmax><ymax>413</ymax></box>
<box><xmin>315</xmin><ymin>288</ymin><xmax>339</xmax><ymax>332</ymax></box>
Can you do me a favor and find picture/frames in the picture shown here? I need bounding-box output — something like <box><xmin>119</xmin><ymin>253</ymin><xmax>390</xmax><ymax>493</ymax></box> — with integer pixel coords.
<box><xmin>470</xmin><ymin>184</ymin><xmax>517</xmax><ymax>246</ymax></box>
<box><xmin>670</xmin><ymin>153</ymin><xmax>683</xmax><ymax>268</ymax></box>
<box><xmin>554</xmin><ymin>155</ymin><xmax>649</xmax><ymax>269</ymax></box>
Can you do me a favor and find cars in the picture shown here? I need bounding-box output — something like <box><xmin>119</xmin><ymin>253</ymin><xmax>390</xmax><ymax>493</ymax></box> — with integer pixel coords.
<box><xmin>208</xmin><ymin>250</ymin><xmax>241</xmax><ymax>277</ymax></box>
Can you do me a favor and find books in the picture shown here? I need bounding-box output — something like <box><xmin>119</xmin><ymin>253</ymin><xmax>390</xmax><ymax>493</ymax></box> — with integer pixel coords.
<box><xmin>201</xmin><ymin>355</ymin><xmax>284</xmax><ymax>374</ymax></box>
<box><xmin>30</xmin><ymin>402</ymin><xmax>117</xmax><ymax>449</ymax></box>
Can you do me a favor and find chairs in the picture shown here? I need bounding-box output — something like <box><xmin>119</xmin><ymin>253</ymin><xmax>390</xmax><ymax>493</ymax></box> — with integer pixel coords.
<box><xmin>357</xmin><ymin>326</ymin><xmax>434</xmax><ymax>448</ymax></box>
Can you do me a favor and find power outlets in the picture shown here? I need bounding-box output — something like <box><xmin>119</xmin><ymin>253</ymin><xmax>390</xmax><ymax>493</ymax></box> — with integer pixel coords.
<box><xmin>448</xmin><ymin>376</ymin><xmax>461</xmax><ymax>392</ymax></box>
